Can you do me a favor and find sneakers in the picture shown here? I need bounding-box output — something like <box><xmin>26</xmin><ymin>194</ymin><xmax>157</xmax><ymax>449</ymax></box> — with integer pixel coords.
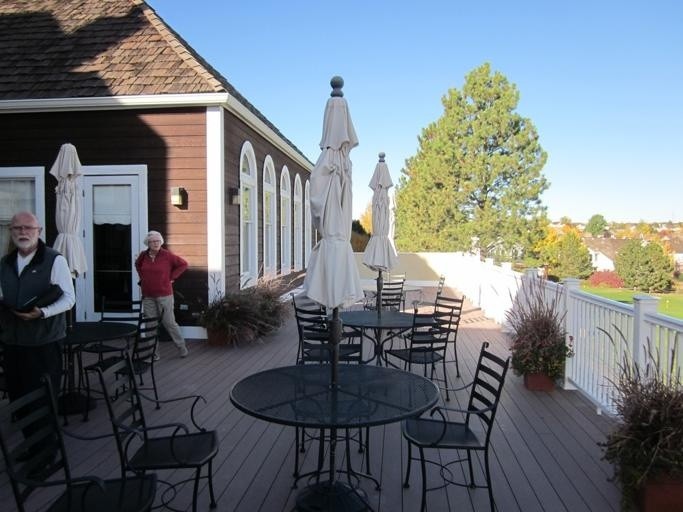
<box><xmin>178</xmin><ymin>344</ymin><xmax>188</xmax><ymax>357</ymax></box>
<box><xmin>152</xmin><ymin>352</ymin><xmax>161</xmax><ymax>363</ymax></box>
<box><xmin>20</xmin><ymin>450</ymin><xmax>57</xmax><ymax>478</ymax></box>
<box><xmin>11</xmin><ymin>440</ymin><xmax>50</xmax><ymax>463</ymax></box>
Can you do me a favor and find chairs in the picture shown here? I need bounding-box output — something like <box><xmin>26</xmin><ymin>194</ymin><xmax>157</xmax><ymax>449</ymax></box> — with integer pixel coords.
<box><xmin>289</xmin><ymin>274</ymin><xmax>466</xmax><ymax>408</ymax></box>
<box><xmin>0</xmin><ymin>372</ymin><xmax>158</xmax><ymax>512</ymax></box>
<box><xmin>94</xmin><ymin>350</ymin><xmax>220</xmax><ymax>512</ymax></box>
<box><xmin>402</xmin><ymin>341</ymin><xmax>513</xmax><ymax>512</ymax></box>
<box><xmin>82</xmin><ymin>307</ymin><xmax>165</xmax><ymax>422</ymax></box>
<box><xmin>72</xmin><ymin>294</ymin><xmax>144</xmax><ymax>403</ymax></box>
<box><xmin>292</xmin><ymin>320</ymin><xmax>372</xmax><ymax>477</ymax></box>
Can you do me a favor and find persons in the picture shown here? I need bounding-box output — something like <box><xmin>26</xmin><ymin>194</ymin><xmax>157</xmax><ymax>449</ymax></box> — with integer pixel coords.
<box><xmin>0</xmin><ymin>210</ymin><xmax>75</xmax><ymax>462</ymax></box>
<box><xmin>133</xmin><ymin>230</ymin><xmax>188</xmax><ymax>365</ymax></box>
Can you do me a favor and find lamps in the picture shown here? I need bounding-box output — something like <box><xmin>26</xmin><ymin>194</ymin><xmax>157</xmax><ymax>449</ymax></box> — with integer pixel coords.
<box><xmin>232</xmin><ymin>187</ymin><xmax>241</xmax><ymax>205</ymax></box>
<box><xmin>170</xmin><ymin>187</ymin><xmax>184</xmax><ymax>206</ymax></box>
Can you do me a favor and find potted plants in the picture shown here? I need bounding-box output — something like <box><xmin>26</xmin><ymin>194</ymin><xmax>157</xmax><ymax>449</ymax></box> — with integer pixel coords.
<box><xmin>195</xmin><ymin>270</ymin><xmax>284</xmax><ymax>348</ymax></box>
<box><xmin>594</xmin><ymin>322</ymin><xmax>683</xmax><ymax>512</ymax></box>
<box><xmin>502</xmin><ymin>265</ymin><xmax>575</xmax><ymax>392</ymax></box>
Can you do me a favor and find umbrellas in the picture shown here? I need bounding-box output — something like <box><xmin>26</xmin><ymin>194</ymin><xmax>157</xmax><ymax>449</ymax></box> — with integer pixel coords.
<box><xmin>360</xmin><ymin>149</ymin><xmax>400</xmax><ymax>315</ymax></box>
<box><xmin>301</xmin><ymin>74</ymin><xmax>365</xmax><ymax>384</ymax></box>
<box><xmin>48</xmin><ymin>142</ymin><xmax>87</xmax><ymax>326</ymax></box>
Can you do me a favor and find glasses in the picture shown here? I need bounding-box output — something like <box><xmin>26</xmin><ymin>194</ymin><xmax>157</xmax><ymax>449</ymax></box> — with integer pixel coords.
<box><xmin>9</xmin><ymin>224</ymin><xmax>41</xmax><ymax>231</ymax></box>
